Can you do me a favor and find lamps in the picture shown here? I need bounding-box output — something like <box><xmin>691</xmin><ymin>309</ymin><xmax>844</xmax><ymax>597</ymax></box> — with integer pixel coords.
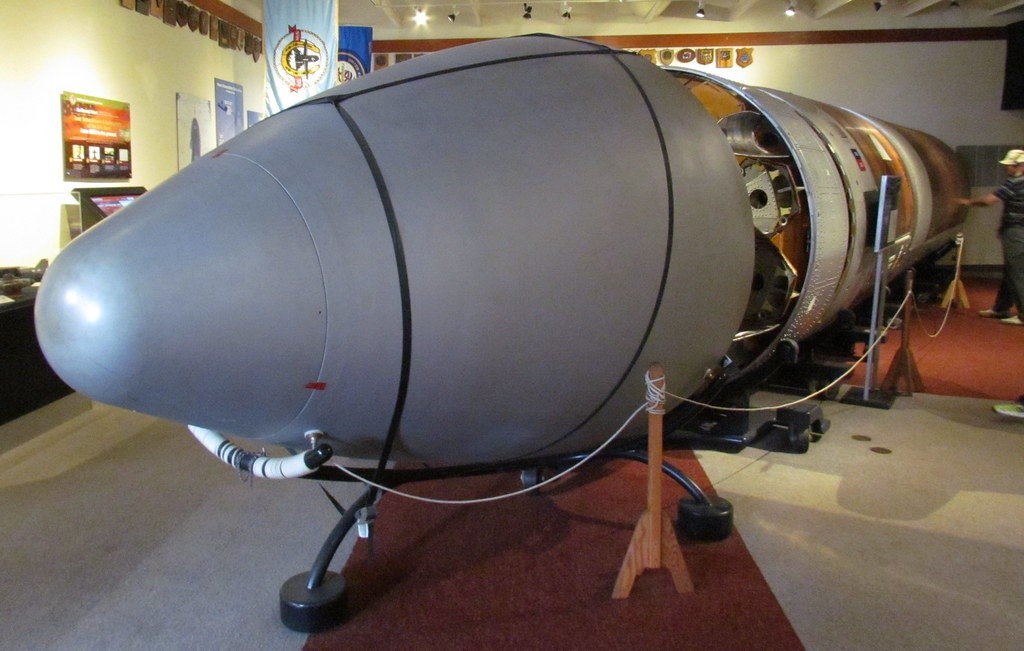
<box><xmin>874</xmin><ymin>0</ymin><xmax>887</xmax><ymax>11</ymax></box>
<box><xmin>787</xmin><ymin>0</ymin><xmax>800</xmax><ymax>16</ymax></box>
<box><xmin>695</xmin><ymin>1</ymin><xmax>704</xmax><ymax>18</ymax></box>
<box><xmin>523</xmin><ymin>3</ymin><xmax>532</xmax><ymax>19</ymax></box>
<box><xmin>448</xmin><ymin>11</ymin><xmax>456</xmax><ymax>21</ymax></box>
<box><xmin>560</xmin><ymin>4</ymin><xmax>571</xmax><ymax>20</ymax></box>
<box><xmin>947</xmin><ymin>0</ymin><xmax>960</xmax><ymax>9</ymax></box>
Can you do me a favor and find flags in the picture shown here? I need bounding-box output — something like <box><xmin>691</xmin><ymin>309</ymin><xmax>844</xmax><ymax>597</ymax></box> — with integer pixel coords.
<box><xmin>338</xmin><ymin>25</ymin><xmax>373</xmax><ymax>84</ymax></box>
<box><xmin>261</xmin><ymin>0</ymin><xmax>338</xmax><ymax>121</ymax></box>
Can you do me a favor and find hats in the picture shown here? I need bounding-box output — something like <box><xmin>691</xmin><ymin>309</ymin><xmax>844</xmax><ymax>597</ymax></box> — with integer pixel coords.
<box><xmin>999</xmin><ymin>149</ymin><xmax>1024</xmax><ymax>165</ymax></box>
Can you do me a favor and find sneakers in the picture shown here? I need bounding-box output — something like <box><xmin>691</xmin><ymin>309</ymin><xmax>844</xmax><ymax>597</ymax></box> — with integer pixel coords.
<box><xmin>992</xmin><ymin>403</ymin><xmax>1024</xmax><ymax>418</ymax></box>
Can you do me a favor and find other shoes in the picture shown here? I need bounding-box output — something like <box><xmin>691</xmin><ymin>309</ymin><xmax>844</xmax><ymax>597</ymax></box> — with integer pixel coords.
<box><xmin>979</xmin><ymin>309</ymin><xmax>1009</xmax><ymax>318</ymax></box>
<box><xmin>1000</xmin><ymin>315</ymin><xmax>1024</xmax><ymax>325</ymax></box>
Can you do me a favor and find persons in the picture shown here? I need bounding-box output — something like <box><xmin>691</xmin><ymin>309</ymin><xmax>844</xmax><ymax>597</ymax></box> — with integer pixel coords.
<box><xmin>950</xmin><ymin>149</ymin><xmax>1024</xmax><ymax>325</ymax></box>
